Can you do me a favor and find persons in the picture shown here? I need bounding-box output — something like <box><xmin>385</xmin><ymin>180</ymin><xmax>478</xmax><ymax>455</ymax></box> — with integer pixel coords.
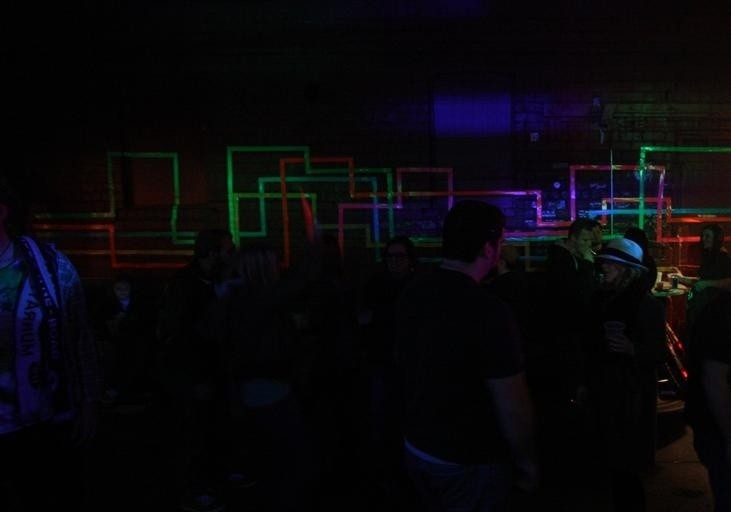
<box><xmin>491</xmin><ymin>219</ymin><xmax>730</xmax><ymax>508</ymax></box>
<box><xmin>388</xmin><ymin>201</ymin><xmax>537</xmax><ymax>509</ymax></box>
<box><xmin>93</xmin><ymin>228</ymin><xmax>433</xmax><ymax>509</ymax></box>
<box><xmin>1</xmin><ymin>206</ymin><xmax>102</xmax><ymax>509</ymax></box>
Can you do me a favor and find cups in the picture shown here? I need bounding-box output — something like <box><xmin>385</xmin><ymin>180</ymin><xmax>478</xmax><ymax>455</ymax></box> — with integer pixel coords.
<box><xmin>657</xmin><ymin>283</ymin><xmax>663</xmax><ymax>290</ymax></box>
<box><xmin>604</xmin><ymin>321</ymin><xmax>621</xmax><ymax>338</ymax></box>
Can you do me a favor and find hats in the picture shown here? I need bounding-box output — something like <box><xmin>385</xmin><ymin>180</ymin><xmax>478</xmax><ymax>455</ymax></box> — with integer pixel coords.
<box><xmin>597</xmin><ymin>238</ymin><xmax>649</xmax><ymax>272</ymax></box>
